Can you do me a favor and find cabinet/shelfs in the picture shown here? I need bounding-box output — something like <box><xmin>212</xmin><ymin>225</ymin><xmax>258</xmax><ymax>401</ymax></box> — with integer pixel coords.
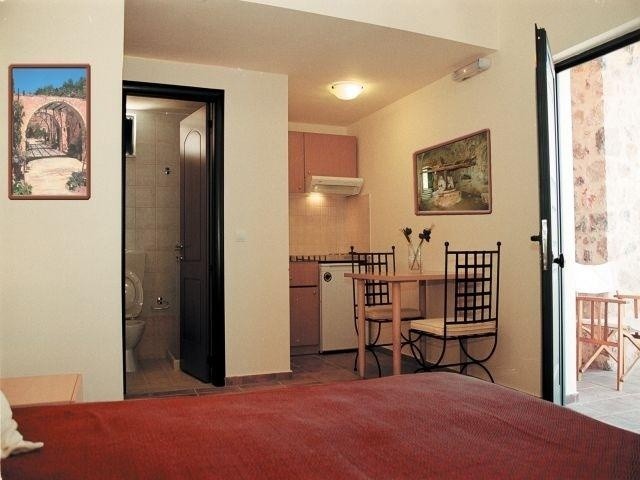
<box><xmin>288</xmin><ymin>131</ymin><xmax>357</xmax><ymax>194</ymax></box>
<box><xmin>290</xmin><ymin>261</ymin><xmax>321</xmax><ymax>356</ymax></box>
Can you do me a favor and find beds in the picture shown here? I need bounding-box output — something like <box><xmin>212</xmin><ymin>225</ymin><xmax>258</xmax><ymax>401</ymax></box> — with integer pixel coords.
<box><xmin>0</xmin><ymin>371</ymin><xmax>640</xmax><ymax>479</ymax></box>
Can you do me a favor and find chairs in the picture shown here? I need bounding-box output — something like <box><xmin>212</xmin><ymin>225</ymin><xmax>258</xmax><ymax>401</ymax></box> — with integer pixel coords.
<box><xmin>576</xmin><ymin>295</ymin><xmax>639</xmax><ymax>391</ymax></box>
<box><xmin>349</xmin><ymin>246</ymin><xmax>426</xmax><ymax>377</ymax></box>
<box><xmin>408</xmin><ymin>242</ymin><xmax>500</xmax><ymax>383</ymax></box>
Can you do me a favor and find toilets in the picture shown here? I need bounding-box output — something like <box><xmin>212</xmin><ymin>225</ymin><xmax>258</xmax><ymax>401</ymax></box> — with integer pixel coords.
<box><xmin>124</xmin><ymin>269</ymin><xmax>148</xmax><ymax>374</ymax></box>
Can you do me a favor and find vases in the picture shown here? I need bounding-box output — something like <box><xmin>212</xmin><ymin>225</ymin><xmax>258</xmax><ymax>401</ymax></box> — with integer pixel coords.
<box><xmin>408</xmin><ymin>243</ymin><xmax>422</xmax><ymax>273</ymax></box>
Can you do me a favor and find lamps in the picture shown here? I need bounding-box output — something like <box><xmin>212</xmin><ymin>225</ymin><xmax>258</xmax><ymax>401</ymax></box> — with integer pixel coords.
<box><xmin>327</xmin><ymin>80</ymin><xmax>366</xmax><ymax>101</ymax></box>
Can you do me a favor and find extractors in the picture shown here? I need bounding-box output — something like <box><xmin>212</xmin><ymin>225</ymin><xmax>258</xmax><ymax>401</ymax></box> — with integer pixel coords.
<box><xmin>311</xmin><ymin>176</ymin><xmax>361</xmax><ymax>195</ymax></box>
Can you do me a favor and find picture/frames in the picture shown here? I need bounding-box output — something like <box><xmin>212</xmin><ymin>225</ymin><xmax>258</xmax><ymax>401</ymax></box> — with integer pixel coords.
<box><xmin>7</xmin><ymin>63</ymin><xmax>92</xmax><ymax>200</ymax></box>
<box><xmin>413</xmin><ymin>128</ymin><xmax>492</xmax><ymax>215</ymax></box>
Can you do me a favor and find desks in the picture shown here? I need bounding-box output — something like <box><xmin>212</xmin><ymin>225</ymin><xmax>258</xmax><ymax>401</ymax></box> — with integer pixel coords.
<box><xmin>343</xmin><ymin>270</ymin><xmax>481</xmax><ymax>379</ymax></box>
